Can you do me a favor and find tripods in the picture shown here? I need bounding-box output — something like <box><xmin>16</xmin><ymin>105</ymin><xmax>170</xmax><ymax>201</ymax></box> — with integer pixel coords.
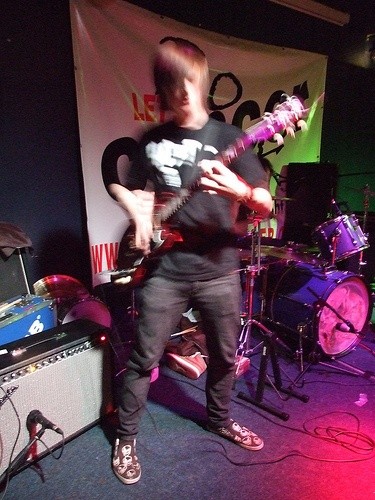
<box><xmin>232</xmin><ymin>216</ymin><xmax>297</xmax><ymax>388</ymax></box>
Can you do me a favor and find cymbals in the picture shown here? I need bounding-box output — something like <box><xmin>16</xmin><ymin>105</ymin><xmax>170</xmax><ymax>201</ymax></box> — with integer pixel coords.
<box><xmin>272</xmin><ymin>196</ymin><xmax>296</xmax><ymax>201</ymax></box>
<box><xmin>32</xmin><ymin>275</ymin><xmax>89</xmax><ymax>304</ymax></box>
<box><xmin>251</xmin><ymin>244</ymin><xmax>326</xmax><ymax>265</ymax></box>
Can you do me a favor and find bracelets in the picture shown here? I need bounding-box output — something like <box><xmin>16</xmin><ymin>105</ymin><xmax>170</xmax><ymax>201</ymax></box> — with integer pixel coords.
<box><xmin>236</xmin><ymin>184</ymin><xmax>254</xmax><ymax>205</ymax></box>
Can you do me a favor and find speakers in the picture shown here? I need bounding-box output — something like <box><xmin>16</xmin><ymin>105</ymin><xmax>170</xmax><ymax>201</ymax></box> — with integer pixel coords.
<box><xmin>0</xmin><ymin>318</ymin><xmax>117</xmax><ymax>482</ymax></box>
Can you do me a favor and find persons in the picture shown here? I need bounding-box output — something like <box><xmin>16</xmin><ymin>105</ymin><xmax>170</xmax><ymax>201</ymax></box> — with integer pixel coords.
<box><xmin>106</xmin><ymin>38</ymin><xmax>273</xmax><ymax>485</ymax></box>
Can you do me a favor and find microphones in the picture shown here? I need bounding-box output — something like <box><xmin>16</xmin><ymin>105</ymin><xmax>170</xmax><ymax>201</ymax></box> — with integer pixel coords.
<box><xmin>28</xmin><ymin>410</ymin><xmax>63</xmax><ymax>433</ymax></box>
<box><xmin>337</xmin><ymin>322</ymin><xmax>354</xmax><ymax>332</ymax></box>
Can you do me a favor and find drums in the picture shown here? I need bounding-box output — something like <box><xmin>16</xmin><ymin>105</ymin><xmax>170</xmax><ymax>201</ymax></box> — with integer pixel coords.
<box><xmin>270</xmin><ymin>263</ymin><xmax>373</xmax><ymax>360</ymax></box>
<box><xmin>238</xmin><ymin>249</ymin><xmax>270</xmax><ymax>318</ymax></box>
<box><xmin>311</xmin><ymin>214</ymin><xmax>371</xmax><ymax>264</ymax></box>
<box><xmin>55</xmin><ymin>295</ymin><xmax>113</xmax><ymax>329</ymax></box>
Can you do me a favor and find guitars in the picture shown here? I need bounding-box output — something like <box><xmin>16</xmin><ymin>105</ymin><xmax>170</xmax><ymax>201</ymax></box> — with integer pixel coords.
<box><xmin>111</xmin><ymin>94</ymin><xmax>310</xmax><ymax>288</ymax></box>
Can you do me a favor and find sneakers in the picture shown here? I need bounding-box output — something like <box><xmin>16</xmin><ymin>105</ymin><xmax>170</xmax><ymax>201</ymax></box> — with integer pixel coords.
<box><xmin>112</xmin><ymin>438</ymin><xmax>141</xmax><ymax>484</ymax></box>
<box><xmin>206</xmin><ymin>418</ymin><xmax>264</xmax><ymax>450</ymax></box>
